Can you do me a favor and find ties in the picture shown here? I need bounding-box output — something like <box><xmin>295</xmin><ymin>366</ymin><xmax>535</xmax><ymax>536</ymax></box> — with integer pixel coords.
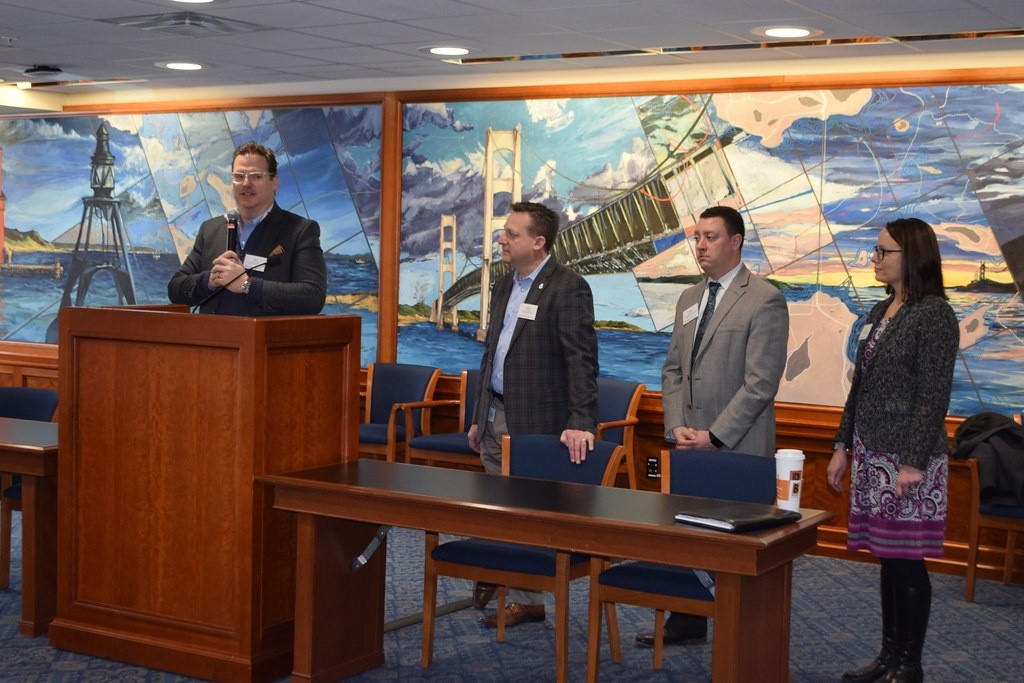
<box><xmin>691</xmin><ymin>281</ymin><xmax>722</xmax><ymax>367</ymax></box>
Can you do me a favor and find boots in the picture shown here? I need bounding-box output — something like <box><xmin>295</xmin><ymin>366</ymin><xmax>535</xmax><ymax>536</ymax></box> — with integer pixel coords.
<box><xmin>874</xmin><ymin>586</ymin><xmax>932</xmax><ymax>683</ymax></box>
<box><xmin>842</xmin><ymin>583</ymin><xmax>905</xmax><ymax>683</ymax></box>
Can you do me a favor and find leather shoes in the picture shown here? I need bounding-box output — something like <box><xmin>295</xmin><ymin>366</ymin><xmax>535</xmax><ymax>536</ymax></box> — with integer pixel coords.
<box><xmin>634</xmin><ymin>626</ymin><xmax>707</xmax><ymax>647</ymax></box>
<box><xmin>479</xmin><ymin>602</ymin><xmax>545</xmax><ymax>628</ymax></box>
<box><xmin>474</xmin><ymin>581</ymin><xmax>497</xmax><ymax>610</ymax></box>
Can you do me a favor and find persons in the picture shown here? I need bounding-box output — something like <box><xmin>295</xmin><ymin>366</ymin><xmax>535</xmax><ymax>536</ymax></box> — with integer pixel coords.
<box><xmin>635</xmin><ymin>206</ymin><xmax>789</xmax><ymax>647</ymax></box>
<box><xmin>467</xmin><ymin>201</ymin><xmax>598</xmax><ymax>626</ymax></box>
<box><xmin>827</xmin><ymin>218</ymin><xmax>961</xmax><ymax>683</ymax></box>
<box><xmin>168</xmin><ymin>142</ymin><xmax>327</xmax><ymax>317</ymax></box>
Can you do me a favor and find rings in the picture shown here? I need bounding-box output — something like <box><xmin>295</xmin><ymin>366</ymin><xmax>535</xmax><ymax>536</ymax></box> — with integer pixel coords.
<box><xmin>581</xmin><ymin>439</ymin><xmax>586</xmax><ymax>442</ymax></box>
<box><xmin>218</xmin><ymin>272</ymin><xmax>220</xmax><ymax>278</ymax></box>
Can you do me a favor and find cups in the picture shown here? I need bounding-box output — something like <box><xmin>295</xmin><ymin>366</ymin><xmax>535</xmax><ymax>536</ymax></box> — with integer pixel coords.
<box><xmin>775</xmin><ymin>448</ymin><xmax>805</xmax><ymax>514</ymax></box>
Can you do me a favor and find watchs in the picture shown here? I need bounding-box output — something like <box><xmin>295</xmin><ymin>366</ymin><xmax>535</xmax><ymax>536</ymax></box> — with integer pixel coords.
<box><xmin>241</xmin><ymin>278</ymin><xmax>250</xmax><ymax>292</ymax></box>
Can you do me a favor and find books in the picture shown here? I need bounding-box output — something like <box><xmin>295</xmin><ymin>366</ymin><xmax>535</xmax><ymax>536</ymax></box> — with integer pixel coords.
<box><xmin>674</xmin><ymin>504</ymin><xmax>802</xmax><ymax>531</ymax></box>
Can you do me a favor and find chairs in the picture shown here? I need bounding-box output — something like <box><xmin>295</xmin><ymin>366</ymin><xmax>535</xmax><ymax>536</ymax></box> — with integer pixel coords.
<box><xmin>588</xmin><ymin>449</ymin><xmax>777</xmax><ymax>683</ymax></box>
<box><xmin>0</xmin><ymin>386</ymin><xmax>60</xmax><ymax>591</ymax></box>
<box><xmin>965</xmin><ymin>456</ymin><xmax>1024</xmax><ymax>607</ymax></box>
<box><xmin>358</xmin><ymin>363</ymin><xmax>443</xmax><ymax>465</ymax></box>
<box><xmin>400</xmin><ymin>370</ymin><xmax>485</xmax><ymax>473</ymax></box>
<box><xmin>595</xmin><ymin>376</ymin><xmax>647</xmax><ymax>491</ymax></box>
<box><xmin>420</xmin><ymin>434</ymin><xmax>628</xmax><ymax>683</ymax></box>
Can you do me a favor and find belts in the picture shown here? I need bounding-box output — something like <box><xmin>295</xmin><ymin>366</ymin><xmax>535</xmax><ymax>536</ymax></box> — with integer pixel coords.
<box><xmin>492</xmin><ymin>390</ymin><xmax>504</xmax><ymax>405</ymax></box>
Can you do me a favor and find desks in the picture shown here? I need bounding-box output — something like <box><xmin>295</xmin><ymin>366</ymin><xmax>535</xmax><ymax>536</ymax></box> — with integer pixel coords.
<box><xmin>262</xmin><ymin>458</ymin><xmax>836</xmax><ymax>683</ymax></box>
<box><xmin>0</xmin><ymin>416</ymin><xmax>57</xmax><ymax>637</ymax></box>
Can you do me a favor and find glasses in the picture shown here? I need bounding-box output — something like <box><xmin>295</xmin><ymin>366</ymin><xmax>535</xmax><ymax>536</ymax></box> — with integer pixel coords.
<box><xmin>231</xmin><ymin>172</ymin><xmax>275</xmax><ymax>184</ymax></box>
<box><xmin>873</xmin><ymin>245</ymin><xmax>902</xmax><ymax>261</ymax></box>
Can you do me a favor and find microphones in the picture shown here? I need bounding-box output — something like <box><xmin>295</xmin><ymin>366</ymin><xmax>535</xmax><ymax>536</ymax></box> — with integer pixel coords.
<box><xmin>226</xmin><ymin>210</ymin><xmax>239</xmax><ymax>258</ymax></box>
<box><xmin>192</xmin><ymin>255</ymin><xmax>281</xmax><ymax>313</ymax></box>
